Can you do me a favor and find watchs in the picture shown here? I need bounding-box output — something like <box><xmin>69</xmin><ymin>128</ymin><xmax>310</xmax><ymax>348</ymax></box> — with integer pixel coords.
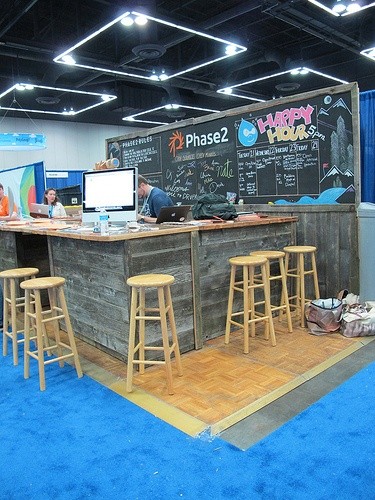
<box><xmin>141</xmin><ymin>215</ymin><xmax>145</xmax><ymax>222</ymax></box>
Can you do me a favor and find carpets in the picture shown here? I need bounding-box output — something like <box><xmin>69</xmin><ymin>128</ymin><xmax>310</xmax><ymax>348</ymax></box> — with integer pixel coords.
<box><xmin>0</xmin><ymin>326</ymin><xmax>375</xmax><ymax>500</ymax></box>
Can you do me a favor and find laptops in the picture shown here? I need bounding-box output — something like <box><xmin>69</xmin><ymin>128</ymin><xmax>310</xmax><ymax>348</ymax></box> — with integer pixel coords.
<box><xmin>30</xmin><ymin>203</ymin><xmax>49</xmax><ymax>218</ymax></box>
<box><xmin>155</xmin><ymin>205</ymin><xmax>190</xmax><ymax>224</ymax></box>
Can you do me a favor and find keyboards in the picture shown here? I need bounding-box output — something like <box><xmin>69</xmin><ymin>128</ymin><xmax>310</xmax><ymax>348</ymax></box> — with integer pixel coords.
<box><xmin>77</xmin><ymin>227</ymin><xmax>124</xmax><ymax>230</ymax></box>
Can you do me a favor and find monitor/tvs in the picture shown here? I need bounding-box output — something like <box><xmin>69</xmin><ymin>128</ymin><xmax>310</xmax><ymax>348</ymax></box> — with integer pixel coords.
<box><xmin>82</xmin><ymin>167</ymin><xmax>138</xmax><ymax>226</ymax></box>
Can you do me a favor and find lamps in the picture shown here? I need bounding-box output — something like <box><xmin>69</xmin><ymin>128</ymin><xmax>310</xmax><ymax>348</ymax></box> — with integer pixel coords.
<box><xmin>0</xmin><ymin>0</ymin><xmax>375</xmax><ymax>125</ymax></box>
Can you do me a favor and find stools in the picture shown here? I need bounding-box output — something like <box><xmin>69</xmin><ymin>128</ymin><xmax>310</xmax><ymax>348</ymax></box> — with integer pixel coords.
<box><xmin>126</xmin><ymin>273</ymin><xmax>183</xmax><ymax>395</ymax></box>
<box><xmin>0</xmin><ymin>268</ymin><xmax>51</xmax><ymax>365</ymax></box>
<box><xmin>278</xmin><ymin>246</ymin><xmax>320</xmax><ymax>328</ymax></box>
<box><xmin>224</xmin><ymin>256</ymin><xmax>276</xmax><ymax>354</ymax></box>
<box><xmin>20</xmin><ymin>276</ymin><xmax>83</xmax><ymax>391</ymax></box>
<box><xmin>250</xmin><ymin>251</ymin><xmax>293</xmax><ymax>340</ymax></box>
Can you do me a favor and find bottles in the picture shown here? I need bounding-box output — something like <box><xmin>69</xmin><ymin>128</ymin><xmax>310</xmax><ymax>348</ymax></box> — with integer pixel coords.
<box><xmin>99</xmin><ymin>207</ymin><xmax>109</xmax><ymax>236</ymax></box>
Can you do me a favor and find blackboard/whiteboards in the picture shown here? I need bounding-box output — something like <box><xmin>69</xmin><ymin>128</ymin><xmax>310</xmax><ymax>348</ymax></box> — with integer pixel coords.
<box><xmin>106</xmin><ymin>82</ymin><xmax>361</xmax><ymax>212</ymax></box>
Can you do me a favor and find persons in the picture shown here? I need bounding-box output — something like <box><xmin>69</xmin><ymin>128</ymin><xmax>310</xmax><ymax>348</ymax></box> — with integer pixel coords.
<box><xmin>138</xmin><ymin>174</ymin><xmax>174</xmax><ymax>223</ymax></box>
<box><xmin>40</xmin><ymin>188</ymin><xmax>66</xmax><ymax>216</ymax></box>
<box><xmin>0</xmin><ymin>183</ymin><xmax>17</xmax><ymax>220</ymax></box>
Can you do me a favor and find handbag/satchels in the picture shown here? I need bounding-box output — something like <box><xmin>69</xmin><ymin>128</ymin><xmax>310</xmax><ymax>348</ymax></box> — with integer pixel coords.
<box><xmin>305</xmin><ymin>297</ymin><xmax>342</xmax><ymax>336</ymax></box>
<box><xmin>191</xmin><ymin>192</ymin><xmax>238</xmax><ymax>220</ymax></box>
<box><xmin>340</xmin><ymin>300</ymin><xmax>375</xmax><ymax>337</ymax></box>
